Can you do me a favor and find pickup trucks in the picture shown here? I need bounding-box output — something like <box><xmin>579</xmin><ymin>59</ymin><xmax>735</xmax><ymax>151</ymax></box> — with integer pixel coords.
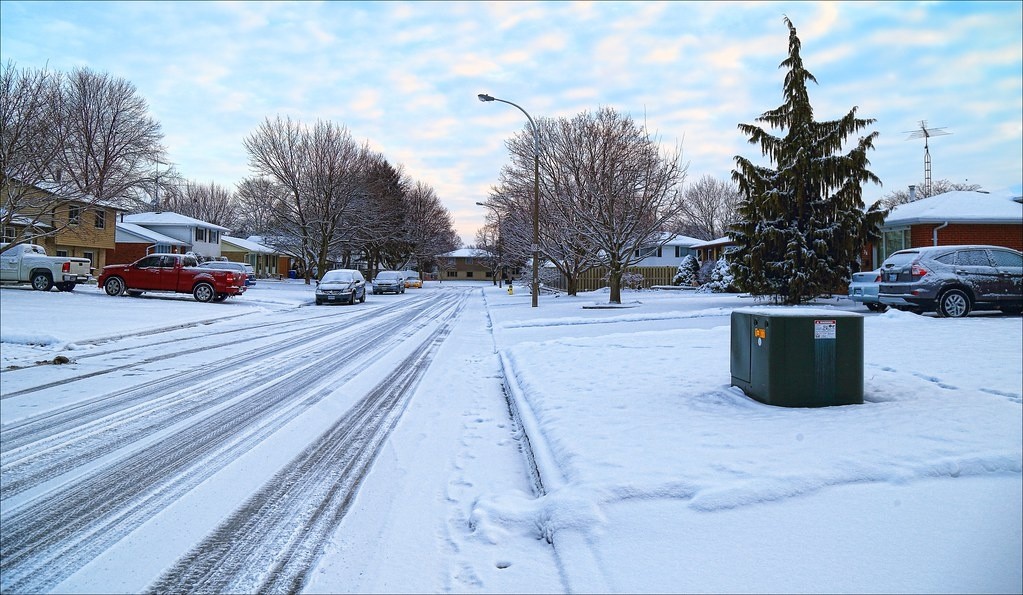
<box><xmin>98</xmin><ymin>253</ymin><xmax>246</xmax><ymax>303</ymax></box>
<box><xmin>0</xmin><ymin>243</ymin><xmax>92</xmax><ymax>293</ymax></box>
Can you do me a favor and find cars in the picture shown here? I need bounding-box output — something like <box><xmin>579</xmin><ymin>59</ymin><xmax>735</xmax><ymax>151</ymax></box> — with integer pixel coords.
<box><xmin>848</xmin><ymin>268</ymin><xmax>924</xmax><ymax>316</ymax></box>
<box><xmin>373</xmin><ymin>271</ymin><xmax>406</xmax><ymax>294</ymax></box>
<box><xmin>405</xmin><ymin>276</ymin><xmax>422</xmax><ymax>288</ymax></box>
<box><xmin>315</xmin><ymin>268</ymin><xmax>365</xmax><ymax>305</ymax></box>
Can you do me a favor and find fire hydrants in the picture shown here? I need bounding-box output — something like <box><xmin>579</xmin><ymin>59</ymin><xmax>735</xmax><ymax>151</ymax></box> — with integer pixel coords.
<box><xmin>508</xmin><ymin>285</ymin><xmax>513</xmax><ymax>295</ymax></box>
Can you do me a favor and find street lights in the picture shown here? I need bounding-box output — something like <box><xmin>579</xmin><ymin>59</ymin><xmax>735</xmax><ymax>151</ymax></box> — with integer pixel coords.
<box><xmin>476</xmin><ymin>202</ymin><xmax>503</xmax><ymax>289</ymax></box>
<box><xmin>478</xmin><ymin>94</ymin><xmax>539</xmax><ymax>307</ymax></box>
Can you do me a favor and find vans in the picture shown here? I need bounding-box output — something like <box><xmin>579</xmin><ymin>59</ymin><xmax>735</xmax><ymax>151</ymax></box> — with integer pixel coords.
<box><xmin>198</xmin><ymin>260</ymin><xmax>256</xmax><ymax>289</ymax></box>
<box><xmin>877</xmin><ymin>245</ymin><xmax>1023</xmax><ymax>318</ymax></box>
